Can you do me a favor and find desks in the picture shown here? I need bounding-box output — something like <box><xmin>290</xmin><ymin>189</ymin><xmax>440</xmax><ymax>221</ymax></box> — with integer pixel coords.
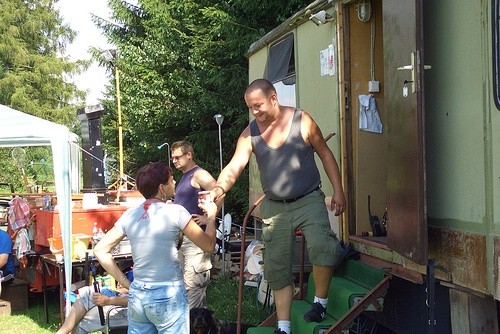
<box><xmin>40</xmin><ymin>240</ymin><xmax>134</xmax><ymax>326</ymax></box>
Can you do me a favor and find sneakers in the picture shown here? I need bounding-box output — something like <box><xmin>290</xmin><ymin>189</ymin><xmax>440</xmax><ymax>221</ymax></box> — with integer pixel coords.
<box><xmin>302</xmin><ymin>299</ymin><xmax>327</xmax><ymax>323</ymax></box>
<box><xmin>273</xmin><ymin>328</ymin><xmax>293</xmax><ymax>334</ymax></box>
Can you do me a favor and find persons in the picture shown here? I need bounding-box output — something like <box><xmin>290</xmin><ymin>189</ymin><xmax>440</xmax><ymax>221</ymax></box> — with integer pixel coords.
<box><xmin>0</xmin><ymin>229</ymin><xmax>15</xmax><ymax>297</ymax></box>
<box><xmin>54</xmin><ymin>266</ymin><xmax>137</xmax><ymax>334</ymax></box>
<box><xmin>92</xmin><ymin>163</ymin><xmax>218</xmax><ymax>334</ymax></box>
<box><xmin>206</xmin><ymin>78</ymin><xmax>348</xmax><ymax>334</ymax></box>
<box><xmin>160</xmin><ymin>140</ymin><xmax>227</xmax><ymax>334</ymax></box>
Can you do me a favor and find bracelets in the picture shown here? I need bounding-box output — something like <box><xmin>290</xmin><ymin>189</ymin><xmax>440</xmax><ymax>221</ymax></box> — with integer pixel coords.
<box><xmin>206</xmin><ymin>217</ymin><xmax>216</xmax><ymax>222</ymax></box>
<box><xmin>213</xmin><ymin>186</ymin><xmax>225</xmax><ymax>195</ymax></box>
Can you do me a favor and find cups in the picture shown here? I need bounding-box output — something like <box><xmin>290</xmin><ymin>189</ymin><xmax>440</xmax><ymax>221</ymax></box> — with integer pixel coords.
<box><xmin>35</xmin><ymin>198</ymin><xmax>44</xmax><ymax>209</ymax></box>
<box><xmin>198</xmin><ymin>191</ymin><xmax>210</xmax><ymax>212</ymax></box>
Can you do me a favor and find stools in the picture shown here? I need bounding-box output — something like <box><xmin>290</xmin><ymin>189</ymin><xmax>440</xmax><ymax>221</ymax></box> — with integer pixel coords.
<box><xmin>0</xmin><ymin>277</ymin><xmax>30</xmax><ymax>312</ymax></box>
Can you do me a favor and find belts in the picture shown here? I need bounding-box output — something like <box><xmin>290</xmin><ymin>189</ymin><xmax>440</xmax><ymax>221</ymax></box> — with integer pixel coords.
<box><xmin>269</xmin><ymin>185</ymin><xmax>319</xmax><ymax>203</ymax></box>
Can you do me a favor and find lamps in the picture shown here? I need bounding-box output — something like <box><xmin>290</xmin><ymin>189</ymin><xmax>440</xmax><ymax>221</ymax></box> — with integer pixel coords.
<box><xmin>309</xmin><ymin>9</ymin><xmax>336</xmax><ymax>27</ymax></box>
<box><xmin>355</xmin><ymin>1</ymin><xmax>373</xmax><ymax>22</ymax></box>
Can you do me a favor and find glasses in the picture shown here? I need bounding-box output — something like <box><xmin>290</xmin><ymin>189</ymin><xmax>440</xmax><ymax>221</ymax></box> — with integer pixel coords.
<box><xmin>169</xmin><ymin>151</ymin><xmax>190</xmax><ymax>161</ymax></box>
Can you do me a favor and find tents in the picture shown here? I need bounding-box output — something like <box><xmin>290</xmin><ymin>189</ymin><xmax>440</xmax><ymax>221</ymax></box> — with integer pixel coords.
<box><xmin>0</xmin><ymin>103</ymin><xmax>82</xmax><ymax>320</ymax></box>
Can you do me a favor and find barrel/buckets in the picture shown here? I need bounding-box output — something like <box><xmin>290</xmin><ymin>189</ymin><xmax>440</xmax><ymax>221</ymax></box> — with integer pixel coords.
<box><xmin>46</xmin><ymin>233</ymin><xmax>90</xmax><ymax>260</ymax></box>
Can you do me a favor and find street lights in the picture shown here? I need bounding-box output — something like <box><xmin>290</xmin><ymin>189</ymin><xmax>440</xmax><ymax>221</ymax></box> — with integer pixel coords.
<box><xmin>214</xmin><ymin>113</ymin><xmax>225</xmax><ymax>277</ymax></box>
<box><xmin>102</xmin><ymin>48</ymin><xmax>124</xmax><ymax>202</ymax></box>
<box><xmin>157</xmin><ymin>142</ymin><xmax>170</xmax><ymax>168</ymax></box>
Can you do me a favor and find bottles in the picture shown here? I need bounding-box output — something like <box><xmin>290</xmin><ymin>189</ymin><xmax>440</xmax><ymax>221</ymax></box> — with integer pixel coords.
<box><xmin>82</xmin><ymin>192</ymin><xmax>98</xmax><ymax>210</ymax></box>
<box><xmin>44</xmin><ymin>195</ymin><xmax>50</xmax><ymax>210</ymax></box>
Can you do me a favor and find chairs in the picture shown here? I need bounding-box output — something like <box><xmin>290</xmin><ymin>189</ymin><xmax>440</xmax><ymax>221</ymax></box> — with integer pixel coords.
<box><xmin>67</xmin><ymin>269</ymin><xmax>134</xmax><ymax>334</ymax></box>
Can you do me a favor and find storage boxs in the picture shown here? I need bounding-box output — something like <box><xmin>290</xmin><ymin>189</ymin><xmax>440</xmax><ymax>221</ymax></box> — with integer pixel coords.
<box><xmin>110</xmin><ymin>241</ymin><xmax>132</xmax><ymax>256</ymax></box>
